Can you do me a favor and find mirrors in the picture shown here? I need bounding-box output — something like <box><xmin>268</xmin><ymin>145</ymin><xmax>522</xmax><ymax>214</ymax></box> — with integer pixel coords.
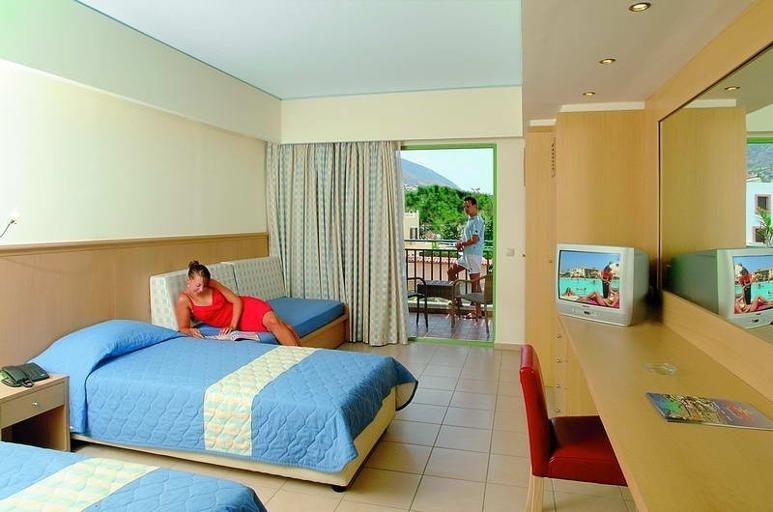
<box><xmin>658</xmin><ymin>45</ymin><xmax>772</xmax><ymax>344</ymax></box>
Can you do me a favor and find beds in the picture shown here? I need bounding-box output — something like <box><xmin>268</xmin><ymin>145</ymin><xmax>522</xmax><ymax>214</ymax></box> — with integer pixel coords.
<box><xmin>0</xmin><ymin>439</ymin><xmax>266</xmax><ymax>511</ymax></box>
<box><xmin>28</xmin><ymin>336</ymin><xmax>396</xmax><ymax>492</ymax></box>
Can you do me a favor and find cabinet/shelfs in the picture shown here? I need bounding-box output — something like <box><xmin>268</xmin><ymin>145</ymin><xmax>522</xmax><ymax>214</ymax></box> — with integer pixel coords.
<box><xmin>661</xmin><ymin>108</ymin><xmax>746</xmax><ymax>294</ymax></box>
<box><xmin>525</xmin><ymin>101</ymin><xmax>646</xmax><ymax>343</ymax></box>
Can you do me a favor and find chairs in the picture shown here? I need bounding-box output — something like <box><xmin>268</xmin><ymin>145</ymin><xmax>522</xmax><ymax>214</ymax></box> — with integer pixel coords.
<box><xmin>451</xmin><ymin>275</ymin><xmax>491</xmax><ymax>335</ymax></box>
<box><xmin>407</xmin><ymin>277</ymin><xmax>428</xmax><ymax>331</ymax></box>
<box><xmin>520</xmin><ymin>344</ymin><xmax>628</xmax><ymax>512</ymax></box>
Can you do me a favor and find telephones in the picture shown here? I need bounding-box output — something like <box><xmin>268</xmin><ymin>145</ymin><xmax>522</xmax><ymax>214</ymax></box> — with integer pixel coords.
<box><xmin>0</xmin><ymin>363</ymin><xmax>49</xmax><ymax>387</ymax></box>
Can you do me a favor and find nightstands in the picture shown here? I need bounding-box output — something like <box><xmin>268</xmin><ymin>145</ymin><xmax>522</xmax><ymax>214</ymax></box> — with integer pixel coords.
<box><xmin>0</xmin><ymin>376</ymin><xmax>70</xmax><ymax>452</ymax></box>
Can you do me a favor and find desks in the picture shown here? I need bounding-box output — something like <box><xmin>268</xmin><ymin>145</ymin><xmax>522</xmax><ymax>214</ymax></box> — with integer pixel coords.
<box><xmin>555</xmin><ymin>316</ymin><xmax>772</xmax><ymax>512</ymax></box>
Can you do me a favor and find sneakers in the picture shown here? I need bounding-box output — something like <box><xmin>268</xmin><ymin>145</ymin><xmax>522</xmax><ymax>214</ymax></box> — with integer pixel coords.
<box><xmin>463</xmin><ymin>312</ymin><xmax>483</xmax><ymax>319</ymax></box>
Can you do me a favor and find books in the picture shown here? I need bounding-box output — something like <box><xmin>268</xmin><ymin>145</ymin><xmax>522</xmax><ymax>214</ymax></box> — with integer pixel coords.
<box><xmin>643</xmin><ymin>393</ymin><xmax>773</xmax><ymax>430</ymax></box>
<box><xmin>205</xmin><ymin>330</ymin><xmax>259</xmax><ymax>342</ymax></box>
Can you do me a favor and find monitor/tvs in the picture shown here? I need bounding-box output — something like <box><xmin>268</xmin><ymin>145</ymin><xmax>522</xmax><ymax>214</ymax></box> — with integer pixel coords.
<box><xmin>554</xmin><ymin>245</ymin><xmax>648</xmax><ymax>327</ymax></box>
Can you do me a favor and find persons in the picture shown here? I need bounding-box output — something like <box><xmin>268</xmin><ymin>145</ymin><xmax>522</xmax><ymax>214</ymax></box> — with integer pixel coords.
<box><xmin>599</xmin><ymin>265</ymin><xmax>613</xmax><ymax>299</ymax></box>
<box><xmin>737</xmin><ymin>293</ymin><xmax>773</xmax><ymax>314</ymax></box>
<box><xmin>174</xmin><ymin>260</ymin><xmax>304</xmax><ymax>348</ymax></box>
<box><xmin>577</xmin><ymin>288</ymin><xmax>619</xmax><ymax>307</ymax></box>
<box><xmin>740</xmin><ymin>266</ymin><xmax>753</xmax><ymax>304</ymax></box>
<box><xmin>447</xmin><ymin>197</ymin><xmax>484</xmax><ymax>319</ymax></box>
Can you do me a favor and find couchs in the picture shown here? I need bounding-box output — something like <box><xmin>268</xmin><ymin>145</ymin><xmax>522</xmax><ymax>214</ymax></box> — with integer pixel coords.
<box><xmin>149</xmin><ymin>256</ymin><xmax>348</xmax><ymax>349</ymax></box>
